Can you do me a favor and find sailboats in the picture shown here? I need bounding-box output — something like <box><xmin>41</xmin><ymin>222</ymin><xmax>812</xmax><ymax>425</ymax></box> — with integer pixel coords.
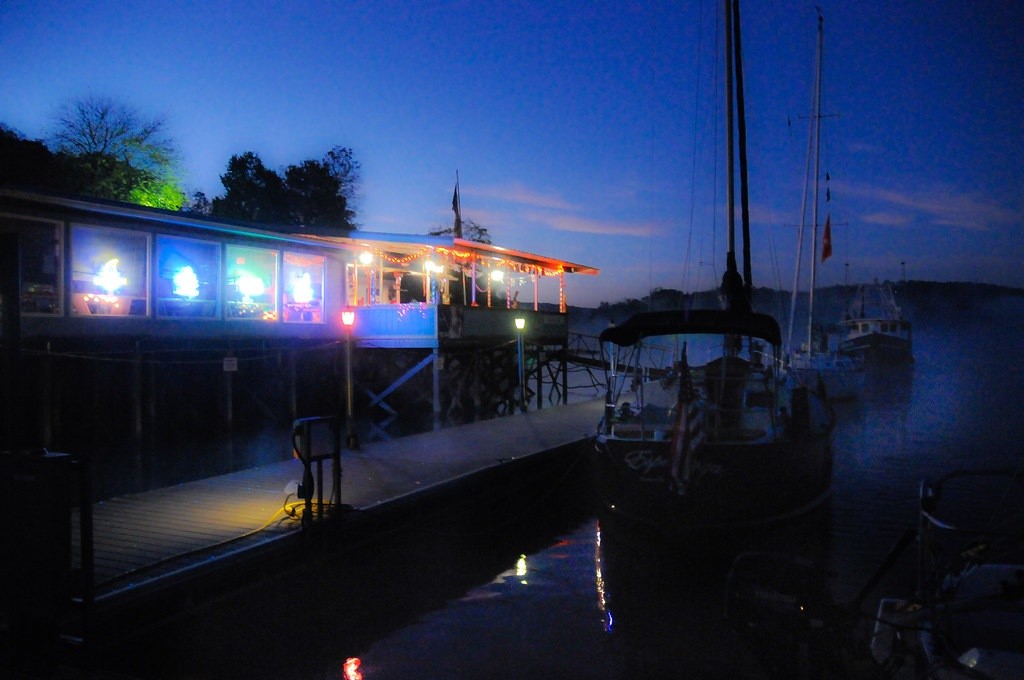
<box><xmin>780</xmin><ymin>10</ymin><xmax>859</xmax><ymax>402</ymax></box>
<box><xmin>593</xmin><ymin>0</ymin><xmax>842</xmax><ymax>560</ymax></box>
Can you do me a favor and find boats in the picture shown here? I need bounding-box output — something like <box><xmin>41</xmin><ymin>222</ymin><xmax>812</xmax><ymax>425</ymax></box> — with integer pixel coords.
<box><xmin>834</xmin><ymin>277</ymin><xmax>916</xmax><ymax>382</ymax></box>
<box><xmin>872</xmin><ymin>454</ymin><xmax>1024</xmax><ymax>679</ymax></box>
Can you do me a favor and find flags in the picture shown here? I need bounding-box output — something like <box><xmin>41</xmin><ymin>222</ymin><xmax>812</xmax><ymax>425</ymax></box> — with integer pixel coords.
<box><xmin>821</xmin><ymin>212</ymin><xmax>831</xmax><ymax>264</ymax></box>
<box><xmin>453</xmin><ymin>188</ymin><xmax>459</xmax><ymax>215</ymax></box>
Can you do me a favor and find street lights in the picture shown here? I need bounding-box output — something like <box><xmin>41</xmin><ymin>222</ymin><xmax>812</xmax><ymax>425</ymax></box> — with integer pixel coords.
<box><xmin>513</xmin><ymin>312</ymin><xmax>528</xmax><ymax>411</ymax></box>
<box><xmin>341</xmin><ymin>299</ymin><xmax>355</xmax><ymax>447</ymax></box>
